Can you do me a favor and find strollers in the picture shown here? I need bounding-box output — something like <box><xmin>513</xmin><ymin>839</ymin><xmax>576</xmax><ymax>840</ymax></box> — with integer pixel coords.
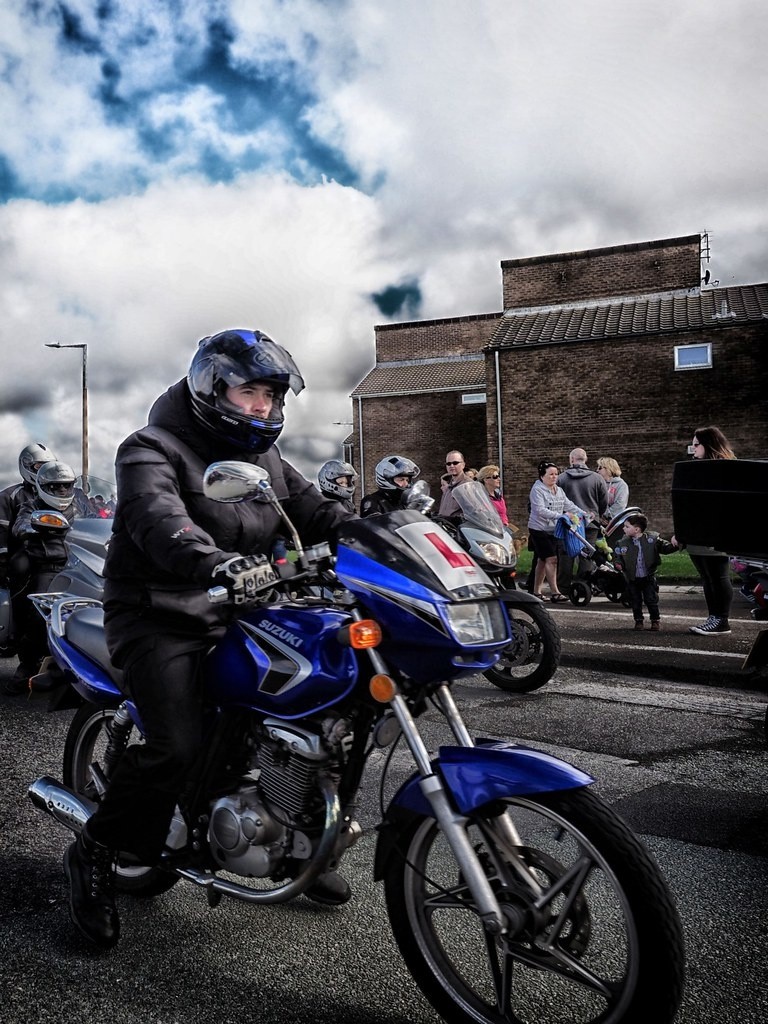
<box><xmin>563</xmin><ymin>507</ymin><xmax>648</xmax><ymax>607</ymax></box>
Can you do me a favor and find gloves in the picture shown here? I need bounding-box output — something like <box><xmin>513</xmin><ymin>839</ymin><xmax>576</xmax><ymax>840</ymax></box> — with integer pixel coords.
<box><xmin>214</xmin><ymin>553</ymin><xmax>278</xmax><ymax>606</ymax></box>
<box><xmin>21</xmin><ymin>528</ymin><xmax>41</xmax><ymax>547</ymax></box>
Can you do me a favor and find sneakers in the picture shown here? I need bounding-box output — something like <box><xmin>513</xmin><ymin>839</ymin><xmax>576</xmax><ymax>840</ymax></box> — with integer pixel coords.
<box><xmin>635</xmin><ymin>619</ymin><xmax>644</xmax><ymax>630</ymax></box>
<box><xmin>739</xmin><ymin>588</ymin><xmax>756</xmax><ymax>603</ymax></box>
<box><xmin>65</xmin><ymin>839</ymin><xmax>122</xmax><ymax>947</ymax></box>
<box><xmin>293</xmin><ymin>862</ymin><xmax>352</xmax><ymax>904</ymax></box>
<box><xmin>695</xmin><ymin>617</ymin><xmax>731</xmax><ymax>636</ymax></box>
<box><xmin>651</xmin><ymin>620</ymin><xmax>660</xmax><ymax>630</ymax></box>
<box><xmin>689</xmin><ymin>614</ymin><xmax>715</xmax><ymax>631</ymax></box>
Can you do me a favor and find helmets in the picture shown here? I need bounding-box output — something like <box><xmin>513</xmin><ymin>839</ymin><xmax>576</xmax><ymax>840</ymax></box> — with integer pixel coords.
<box><xmin>376</xmin><ymin>456</ymin><xmax>421</xmax><ymax>493</ymax></box>
<box><xmin>37</xmin><ymin>461</ymin><xmax>76</xmax><ymax>511</ymax></box>
<box><xmin>19</xmin><ymin>442</ymin><xmax>53</xmax><ymax>486</ymax></box>
<box><xmin>188</xmin><ymin>330</ymin><xmax>305</xmax><ymax>454</ymax></box>
<box><xmin>318</xmin><ymin>460</ymin><xmax>359</xmax><ymax>498</ymax></box>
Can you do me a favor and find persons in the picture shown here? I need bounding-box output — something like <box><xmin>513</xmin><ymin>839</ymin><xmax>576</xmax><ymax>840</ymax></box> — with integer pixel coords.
<box><xmin>0</xmin><ymin>444</ymin><xmax>115</xmax><ymax>694</ymax></box>
<box><xmin>672</xmin><ymin>424</ymin><xmax>740</xmax><ymax>635</ymax></box>
<box><xmin>524</xmin><ymin>463</ymin><xmax>596</xmax><ymax>604</ymax></box>
<box><xmin>319</xmin><ymin>457</ymin><xmax>359</xmax><ymax>515</ymax></box>
<box><xmin>477</xmin><ymin>463</ymin><xmax>516</xmax><ymax>590</ymax></box>
<box><xmin>438</xmin><ymin>450</ymin><xmax>481</xmax><ymax>516</ymax></box>
<box><xmin>555</xmin><ymin>446</ymin><xmax>608</xmax><ymax>597</ymax></box>
<box><xmin>612</xmin><ymin>512</ymin><xmax>681</xmax><ymax>629</ymax></box>
<box><xmin>359</xmin><ymin>453</ymin><xmax>421</xmax><ymax>518</ymax></box>
<box><xmin>593</xmin><ymin>456</ymin><xmax>630</xmax><ymax>582</ymax></box>
<box><xmin>53</xmin><ymin>329</ymin><xmax>352</xmax><ymax>948</ymax></box>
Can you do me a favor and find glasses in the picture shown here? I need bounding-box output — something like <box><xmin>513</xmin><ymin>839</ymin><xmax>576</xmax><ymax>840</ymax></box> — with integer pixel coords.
<box><xmin>695</xmin><ymin>442</ymin><xmax>701</xmax><ymax>447</ymax></box>
<box><xmin>545</xmin><ymin>462</ymin><xmax>555</xmax><ymax>466</ymax></box>
<box><xmin>487</xmin><ymin>474</ymin><xmax>500</xmax><ymax>480</ymax></box>
<box><xmin>599</xmin><ymin>466</ymin><xmax>606</xmax><ymax>470</ymax></box>
<box><xmin>49</xmin><ymin>482</ymin><xmax>72</xmax><ymax>491</ymax></box>
<box><xmin>446</xmin><ymin>461</ymin><xmax>460</xmax><ymax>466</ymax></box>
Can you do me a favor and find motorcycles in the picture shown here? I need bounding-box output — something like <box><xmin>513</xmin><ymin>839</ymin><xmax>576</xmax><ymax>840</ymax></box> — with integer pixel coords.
<box><xmin>670</xmin><ymin>459</ymin><xmax>768</xmax><ymax>671</ymax></box>
<box><xmin>1</xmin><ymin>509</ymin><xmax>117</xmax><ymax>692</ymax></box>
<box><xmin>26</xmin><ymin>458</ymin><xmax>686</xmax><ymax>1024</ymax></box>
<box><xmin>401</xmin><ymin>483</ymin><xmax>562</xmax><ymax>692</ymax></box>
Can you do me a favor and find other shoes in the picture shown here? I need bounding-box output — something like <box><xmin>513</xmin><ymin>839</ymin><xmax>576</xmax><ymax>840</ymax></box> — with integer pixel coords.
<box><xmin>518</xmin><ymin>581</ymin><xmax>529</xmax><ymax>590</ymax></box>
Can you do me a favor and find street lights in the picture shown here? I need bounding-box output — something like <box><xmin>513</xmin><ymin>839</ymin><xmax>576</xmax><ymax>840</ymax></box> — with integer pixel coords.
<box><xmin>45</xmin><ymin>342</ymin><xmax>89</xmax><ymax>490</ymax></box>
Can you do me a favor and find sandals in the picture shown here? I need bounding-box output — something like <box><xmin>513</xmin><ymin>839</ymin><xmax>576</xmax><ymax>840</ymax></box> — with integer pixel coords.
<box><xmin>550</xmin><ymin>593</ymin><xmax>569</xmax><ymax>603</ymax></box>
<box><xmin>534</xmin><ymin>593</ymin><xmax>550</xmax><ymax>601</ymax></box>
